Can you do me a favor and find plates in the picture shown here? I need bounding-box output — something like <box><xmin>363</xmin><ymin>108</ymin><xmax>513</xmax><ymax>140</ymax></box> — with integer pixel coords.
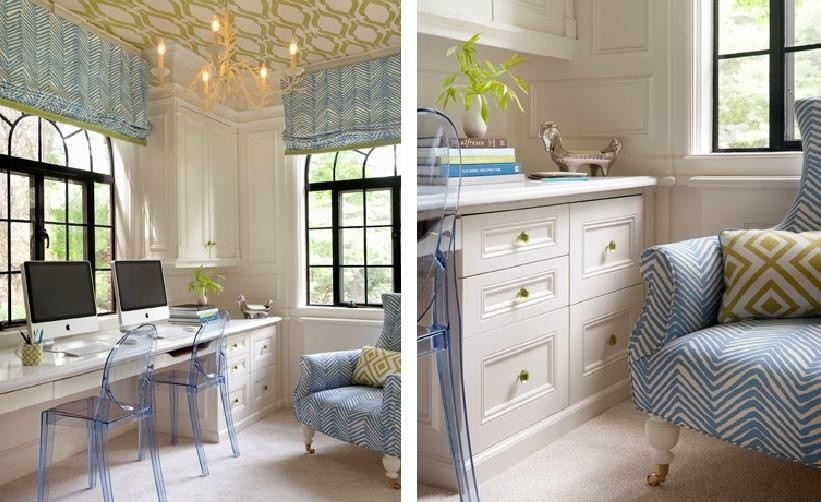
<box><xmin>528</xmin><ymin>172</ymin><xmax>588</xmax><ymax>179</ymax></box>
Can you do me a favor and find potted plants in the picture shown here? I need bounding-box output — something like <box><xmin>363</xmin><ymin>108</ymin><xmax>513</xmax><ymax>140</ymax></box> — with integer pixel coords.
<box><xmin>181</xmin><ymin>266</ymin><xmax>227</xmax><ymax>305</ymax></box>
<box><xmin>436</xmin><ymin>29</ymin><xmax>532</xmax><ymax>137</ymax></box>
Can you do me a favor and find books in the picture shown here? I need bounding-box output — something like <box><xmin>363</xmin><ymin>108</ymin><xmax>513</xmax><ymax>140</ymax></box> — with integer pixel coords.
<box><xmin>168</xmin><ymin>304</ymin><xmax>222</xmax><ymax>327</ymax></box>
<box><xmin>417</xmin><ymin>136</ymin><xmax>526</xmax><ymax>187</ymax></box>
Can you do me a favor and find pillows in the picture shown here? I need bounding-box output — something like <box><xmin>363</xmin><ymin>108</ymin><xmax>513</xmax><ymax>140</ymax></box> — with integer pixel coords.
<box><xmin>343</xmin><ymin>342</ymin><xmax>400</xmax><ymax>386</ymax></box>
<box><xmin>710</xmin><ymin>224</ymin><xmax>821</xmax><ymax>323</ymax></box>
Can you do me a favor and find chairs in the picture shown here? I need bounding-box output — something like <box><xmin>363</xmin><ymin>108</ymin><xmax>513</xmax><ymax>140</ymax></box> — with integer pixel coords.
<box><xmin>626</xmin><ymin>99</ymin><xmax>821</xmax><ymax>497</ymax></box>
<box><xmin>291</xmin><ymin>294</ymin><xmax>400</xmax><ymax>490</ymax></box>
<box><xmin>138</xmin><ymin>309</ymin><xmax>241</xmax><ymax>478</ymax></box>
<box><xmin>35</xmin><ymin>322</ymin><xmax>168</xmax><ymax>501</ymax></box>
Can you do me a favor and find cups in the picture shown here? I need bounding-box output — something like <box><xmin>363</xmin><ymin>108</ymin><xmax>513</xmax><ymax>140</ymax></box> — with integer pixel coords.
<box><xmin>14</xmin><ymin>343</ymin><xmax>43</xmax><ymax>365</ymax></box>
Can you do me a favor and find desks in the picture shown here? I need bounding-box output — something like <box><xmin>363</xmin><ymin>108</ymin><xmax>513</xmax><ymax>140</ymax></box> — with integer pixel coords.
<box><xmin>0</xmin><ymin>314</ymin><xmax>282</xmax><ymax>445</ymax></box>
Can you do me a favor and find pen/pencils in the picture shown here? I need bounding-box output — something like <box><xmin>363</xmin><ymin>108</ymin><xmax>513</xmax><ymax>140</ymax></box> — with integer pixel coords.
<box><xmin>20</xmin><ymin>328</ymin><xmax>44</xmax><ymax>344</ymax></box>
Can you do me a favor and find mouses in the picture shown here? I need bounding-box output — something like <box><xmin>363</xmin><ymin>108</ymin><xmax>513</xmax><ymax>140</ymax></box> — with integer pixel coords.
<box><xmin>184</xmin><ymin>327</ymin><xmax>195</xmax><ymax>332</ymax></box>
<box><xmin>122</xmin><ymin>338</ymin><xmax>137</xmax><ymax>344</ymax></box>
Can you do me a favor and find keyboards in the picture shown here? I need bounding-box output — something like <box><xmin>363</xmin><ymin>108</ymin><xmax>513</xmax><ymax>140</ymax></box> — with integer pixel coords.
<box><xmin>146</xmin><ymin>329</ymin><xmax>183</xmax><ymax>339</ymax></box>
<box><xmin>63</xmin><ymin>342</ymin><xmax>110</xmax><ymax>357</ymax></box>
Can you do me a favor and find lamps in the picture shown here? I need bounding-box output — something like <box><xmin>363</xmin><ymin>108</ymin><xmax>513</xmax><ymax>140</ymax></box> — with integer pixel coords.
<box><xmin>145</xmin><ymin>1</ymin><xmax>305</xmax><ymax>116</ymax></box>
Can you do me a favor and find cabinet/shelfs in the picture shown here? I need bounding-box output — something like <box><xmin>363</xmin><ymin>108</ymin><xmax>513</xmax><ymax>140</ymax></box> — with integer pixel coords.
<box><xmin>136</xmin><ymin>97</ymin><xmax>241</xmax><ymax>268</ymax></box>
<box><xmin>417</xmin><ymin>195</ymin><xmax>659</xmax><ymax>492</ymax></box>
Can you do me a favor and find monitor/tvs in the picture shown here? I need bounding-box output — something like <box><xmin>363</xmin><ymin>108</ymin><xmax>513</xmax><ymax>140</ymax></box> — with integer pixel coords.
<box><xmin>20</xmin><ymin>259</ymin><xmax>99</xmax><ymax>353</ymax></box>
<box><xmin>111</xmin><ymin>259</ymin><xmax>170</xmax><ymax>333</ymax></box>
